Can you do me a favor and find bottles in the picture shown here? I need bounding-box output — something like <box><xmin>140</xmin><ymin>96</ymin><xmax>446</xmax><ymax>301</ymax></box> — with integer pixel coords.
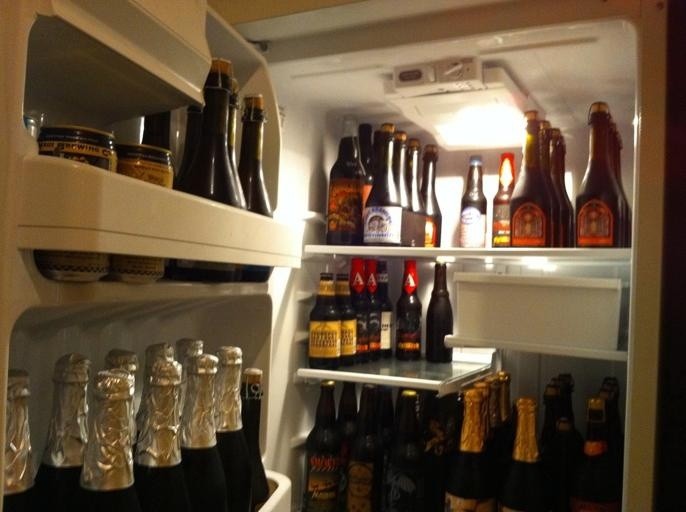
<box><xmin>494</xmin><ymin>151</ymin><xmax>515</xmax><ymax>245</ymax></box>
<box><xmin>323</xmin><ymin>111</ymin><xmax>444</xmax><ymax>247</ymax></box>
<box><xmin>298</xmin><ymin>373</ymin><xmax>626</xmax><ymax>511</ymax></box>
<box><xmin>508</xmin><ymin>111</ymin><xmax>574</xmax><ymax>248</ymax></box>
<box><xmin>571</xmin><ymin>102</ymin><xmax>633</xmax><ymax>249</ymax></box>
<box><xmin>427</xmin><ymin>263</ymin><xmax>456</xmax><ymax>363</ymax></box>
<box><xmin>141</xmin><ymin>57</ymin><xmax>276</xmax><ymax>286</ymax></box>
<box><xmin>2</xmin><ymin>333</ymin><xmax>264</xmax><ymax>512</ymax></box>
<box><xmin>307</xmin><ymin>259</ymin><xmax>392</xmax><ymax>369</ymax></box>
<box><xmin>396</xmin><ymin>259</ymin><xmax>421</xmax><ymax>360</ymax></box>
<box><xmin>459</xmin><ymin>153</ymin><xmax>489</xmax><ymax>248</ymax></box>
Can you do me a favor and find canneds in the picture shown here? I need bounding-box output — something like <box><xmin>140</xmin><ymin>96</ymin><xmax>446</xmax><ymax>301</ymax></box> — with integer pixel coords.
<box><xmin>38</xmin><ymin>125</ymin><xmax>118</xmax><ymax>282</ymax></box>
<box><xmin>107</xmin><ymin>141</ymin><xmax>173</xmax><ymax>283</ymax></box>
